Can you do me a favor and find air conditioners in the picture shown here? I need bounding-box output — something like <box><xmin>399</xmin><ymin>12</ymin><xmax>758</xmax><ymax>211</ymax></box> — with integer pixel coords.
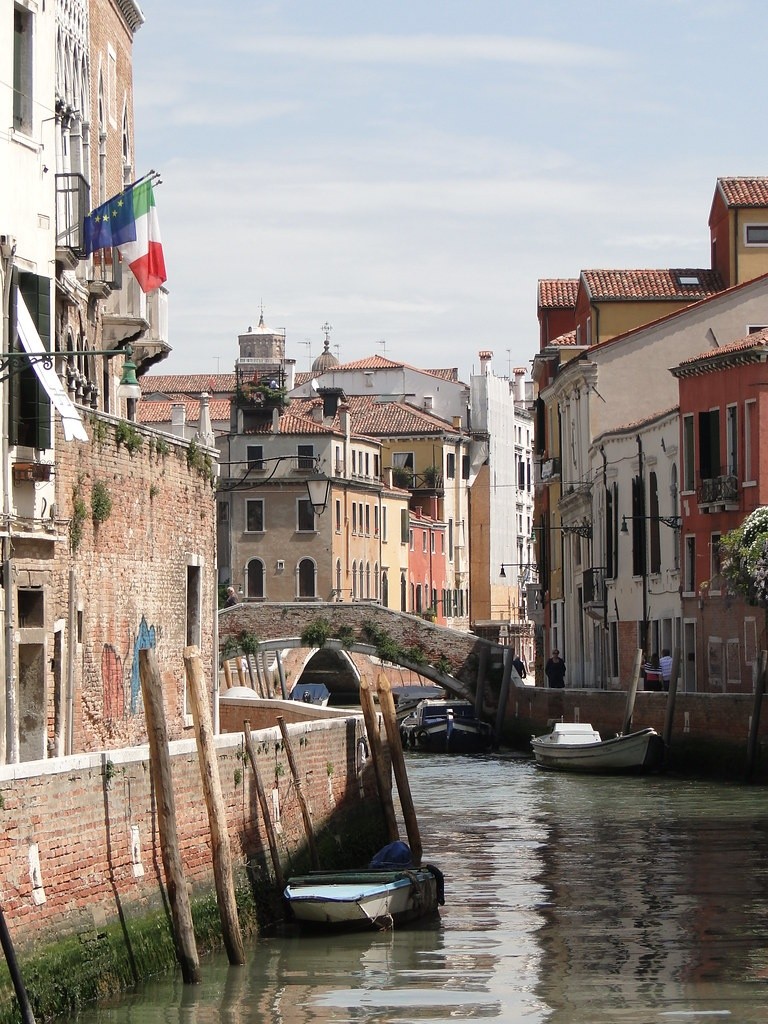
<box><xmin>581</xmin><ymin>567</ymin><xmax>608</xmax><ymax>602</ymax></box>
<box><xmin>702</xmin><ymin>479</ymin><xmax>713</xmax><ymax>502</ymax></box>
<box><xmin>716</xmin><ymin>475</ymin><xmax>738</xmax><ymax>500</ymax></box>
<box><xmin>541</xmin><ymin>459</ymin><xmax>560</xmax><ymax>478</ymax></box>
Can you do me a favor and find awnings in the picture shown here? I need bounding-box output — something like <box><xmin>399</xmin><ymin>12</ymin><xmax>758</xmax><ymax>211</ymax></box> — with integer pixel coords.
<box><xmin>17</xmin><ymin>287</ymin><xmax>89</xmax><ymax>442</ymax></box>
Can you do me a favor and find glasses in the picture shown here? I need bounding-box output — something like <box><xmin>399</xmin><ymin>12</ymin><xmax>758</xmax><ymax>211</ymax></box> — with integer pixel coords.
<box><xmin>554</xmin><ymin>653</ymin><xmax>558</xmax><ymax>654</ymax></box>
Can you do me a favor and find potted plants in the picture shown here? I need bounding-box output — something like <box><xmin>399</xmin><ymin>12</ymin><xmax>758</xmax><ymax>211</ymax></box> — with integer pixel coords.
<box><xmin>393</xmin><ymin>466</ymin><xmax>414</xmax><ymax>489</ymax></box>
<box><xmin>260</xmin><ymin>385</ymin><xmax>287</xmax><ymax>406</ymax></box>
<box><xmin>421</xmin><ymin>607</ymin><xmax>436</xmax><ymax>622</ymax></box>
<box><xmin>424</xmin><ymin>465</ymin><xmax>440</xmax><ymax>488</ymax></box>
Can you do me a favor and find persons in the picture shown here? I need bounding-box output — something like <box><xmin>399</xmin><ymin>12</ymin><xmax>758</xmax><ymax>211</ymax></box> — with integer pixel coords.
<box><xmin>641</xmin><ymin>649</ymin><xmax>673</xmax><ymax>692</ymax></box>
<box><xmin>546</xmin><ymin>650</ymin><xmax>566</xmax><ymax>688</ymax></box>
<box><xmin>513</xmin><ymin>656</ymin><xmax>526</xmax><ymax>680</ymax></box>
<box><xmin>224</xmin><ymin>587</ymin><xmax>239</xmax><ymax>609</ymax></box>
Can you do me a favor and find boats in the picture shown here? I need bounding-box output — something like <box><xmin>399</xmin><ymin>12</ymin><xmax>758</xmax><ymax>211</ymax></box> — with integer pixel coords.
<box><xmin>286</xmin><ymin>683</ymin><xmax>330</xmax><ymax>710</ymax></box>
<box><xmin>401</xmin><ymin>699</ymin><xmax>493</xmax><ymax>754</ymax></box>
<box><xmin>285</xmin><ymin>842</ymin><xmax>445</xmax><ymax>938</ymax></box>
<box><xmin>531</xmin><ymin>715</ymin><xmax>657</xmax><ymax>775</ymax></box>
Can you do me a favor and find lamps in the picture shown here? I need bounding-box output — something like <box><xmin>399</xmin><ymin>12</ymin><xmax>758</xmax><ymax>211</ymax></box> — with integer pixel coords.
<box><xmin>305</xmin><ymin>460</ymin><xmax>329</xmax><ymax>517</ymax></box>
<box><xmin>619</xmin><ymin>514</ymin><xmax>681</xmax><ymax>536</ymax></box>
<box><xmin>0</xmin><ymin>345</ymin><xmax>143</xmax><ymax>400</ymax></box>
<box><xmin>499</xmin><ymin>563</ymin><xmax>537</xmax><ymax>577</ymax></box>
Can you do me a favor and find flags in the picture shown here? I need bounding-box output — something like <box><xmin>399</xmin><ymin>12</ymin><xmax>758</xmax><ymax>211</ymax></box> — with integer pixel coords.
<box><xmin>116</xmin><ymin>179</ymin><xmax>166</xmax><ymax>293</ymax></box>
<box><xmin>83</xmin><ymin>177</ymin><xmax>143</xmax><ymax>254</ymax></box>
